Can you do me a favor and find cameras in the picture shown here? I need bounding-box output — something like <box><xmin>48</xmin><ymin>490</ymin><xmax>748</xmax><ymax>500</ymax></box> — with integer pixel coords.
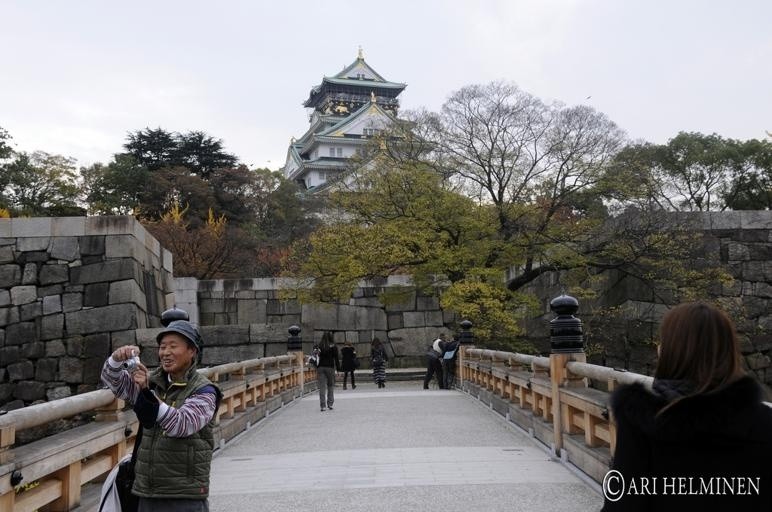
<box><xmin>122</xmin><ymin>349</ymin><xmax>141</xmax><ymax>379</ymax></box>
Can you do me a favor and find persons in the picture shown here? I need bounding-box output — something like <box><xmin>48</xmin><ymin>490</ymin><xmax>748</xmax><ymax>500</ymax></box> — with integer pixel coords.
<box><xmin>599</xmin><ymin>303</ymin><xmax>772</xmax><ymax>512</ymax></box>
<box><xmin>340</xmin><ymin>340</ymin><xmax>357</xmax><ymax>390</ymax></box>
<box><xmin>100</xmin><ymin>319</ymin><xmax>223</xmax><ymax>512</ymax></box>
<box><xmin>443</xmin><ymin>336</ymin><xmax>459</xmax><ymax>389</ymax></box>
<box><xmin>316</xmin><ymin>330</ymin><xmax>340</xmax><ymax>411</ymax></box>
<box><xmin>369</xmin><ymin>337</ymin><xmax>388</xmax><ymax>388</ymax></box>
<box><xmin>423</xmin><ymin>333</ymin><xmax>445</xmax><ymax>389</ymax></box>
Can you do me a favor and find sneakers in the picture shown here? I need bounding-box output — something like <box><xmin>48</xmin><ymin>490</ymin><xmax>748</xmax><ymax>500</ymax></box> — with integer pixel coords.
<box><xmin>320</xmin><ymin>404</ymin><xmax>334</xmax><ymax>412</ymax></box>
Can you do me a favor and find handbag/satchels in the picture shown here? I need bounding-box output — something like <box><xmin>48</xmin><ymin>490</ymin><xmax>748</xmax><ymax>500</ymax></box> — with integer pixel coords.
<box><xmin>425</xmin><ymin>349</ymin><xmax>440</xmax><ymax>362</ymax></box>
<box><xmin>443</xmin><ymin>351</ymin><xmax>455</xmax><ymax>360</ymax></box>
<box><xmin>306</xmin><ymin>348</ymin><xmax>320</xmax><ymax>367</ymax></box>
<box><xmin>113</xmin><ymin>458</ymin><xmax>139</xmax><ymax>512</ymax></box>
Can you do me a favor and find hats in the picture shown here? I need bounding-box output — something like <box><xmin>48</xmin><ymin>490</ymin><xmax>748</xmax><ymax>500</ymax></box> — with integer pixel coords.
<box><xmin>157</xmin><ymin>320</ymin><xmax>203</xmax><ymax>355</ymax></box>
